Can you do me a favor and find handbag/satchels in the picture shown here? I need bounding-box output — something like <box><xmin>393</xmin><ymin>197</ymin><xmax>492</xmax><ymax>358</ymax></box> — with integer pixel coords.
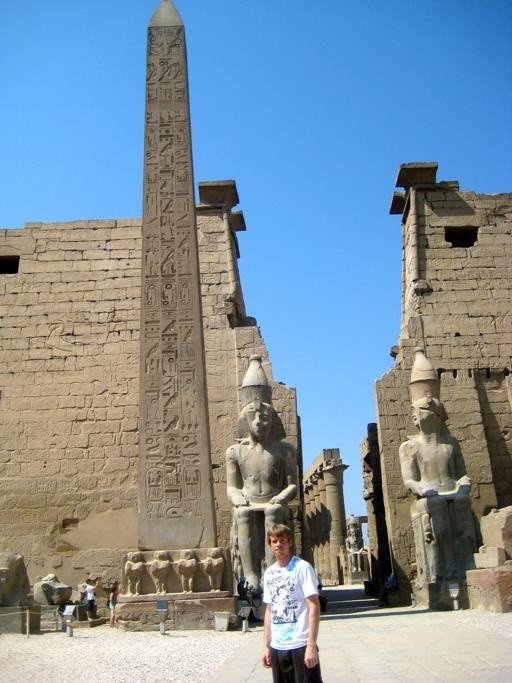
<box><xmin>106</xmin><ymin>598</ymin><xmax>110</xmax><ymax>606</ymax></box>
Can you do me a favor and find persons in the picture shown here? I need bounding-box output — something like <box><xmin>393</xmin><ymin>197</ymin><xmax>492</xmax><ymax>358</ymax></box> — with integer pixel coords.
<box><xmin>318</xmin><ymin>583</ymin><xmax>328</xmax><ymax>611</ymax></box>
<box><xmin>86</xmin><ymin>578</ymin><xmax>99</xmax><ymax>619</ymax></box>
<box><xmin>345</xmin><ymin>524</ymin><xmax>363</xmax><ymax>572</ymax></box>
<box><xmin>108</xmin><ymin>585</ymin><xmax>116</xmax><ymax>627</ymax></box>
<box><xmin>226</xmin><ymin>400</ymin><xmax>298</xmax><ymax>592</ymax></box>
<box><xmin>262</xmin><ymin>524</ymin><xmax>323</xmax><ymax>682</ymax></box>
<box><xmin>237</xmin><ymin>574</ymin><xmax>258</xmax><ymax>608</ymax></box>
<box><xmin>398</xmin><ymin>397</ymin><xmax>479</xmax><ymax>581</ymax></box>
